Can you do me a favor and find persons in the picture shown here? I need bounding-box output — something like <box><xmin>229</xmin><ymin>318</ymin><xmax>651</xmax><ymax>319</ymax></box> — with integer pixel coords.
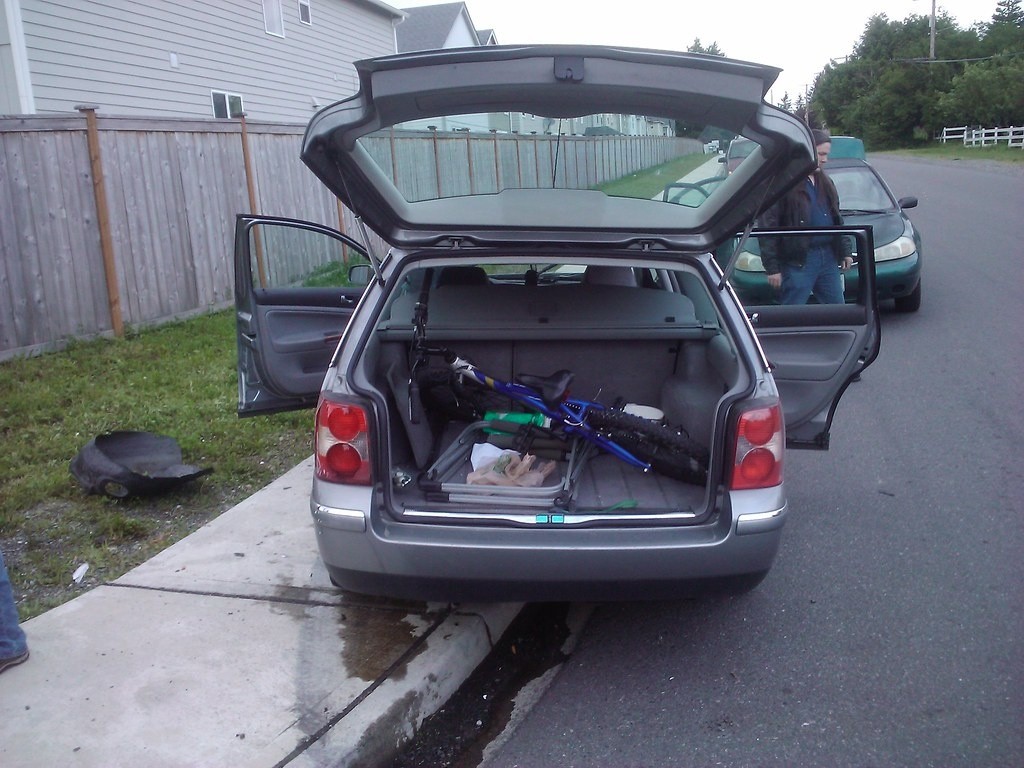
<box><xmin>758</xmin><ymin>129</ymin><xmax>863</xmax><ymax>308</ymax></box>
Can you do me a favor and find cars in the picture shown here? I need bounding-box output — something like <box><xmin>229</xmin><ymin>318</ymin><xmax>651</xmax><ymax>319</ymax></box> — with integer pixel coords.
<box><xmin>719</xmin><ymin>136</ymin><xmax>921</xmax><ymax>315</ymax></box>
<box><xmin>229</xmin><ymin>42</ymin><xmax>883</xmax><ymax>601</ymax></box>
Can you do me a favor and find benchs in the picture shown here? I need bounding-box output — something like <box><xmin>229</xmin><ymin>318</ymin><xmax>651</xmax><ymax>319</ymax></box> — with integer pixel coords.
<box><xmin>389</xmin><ymin>283</ymin><xmax>698</xmax><ymax>422</ymax></box>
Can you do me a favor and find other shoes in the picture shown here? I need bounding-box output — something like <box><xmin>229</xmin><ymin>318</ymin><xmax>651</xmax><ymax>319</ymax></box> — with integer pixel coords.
<box><xmin>0</xmin><ymin>650</ymin><xmax>30</xmax><ymax>673</ymax></box>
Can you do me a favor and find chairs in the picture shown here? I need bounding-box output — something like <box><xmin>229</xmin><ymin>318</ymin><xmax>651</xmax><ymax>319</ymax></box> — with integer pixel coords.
<box><xmin>836</xmin><ymin>180</ymin><xmax>860</xmax><ymax>201</ymax></box>
<box><xmin>438</xmin><ymin>268</ymin><xmax>489</xmax><ymax>284</ymax></box>
<box><xmin>582</xmin><ymin>265</ymin><xmax>638</xmax><ymax>287</ymax></box>
